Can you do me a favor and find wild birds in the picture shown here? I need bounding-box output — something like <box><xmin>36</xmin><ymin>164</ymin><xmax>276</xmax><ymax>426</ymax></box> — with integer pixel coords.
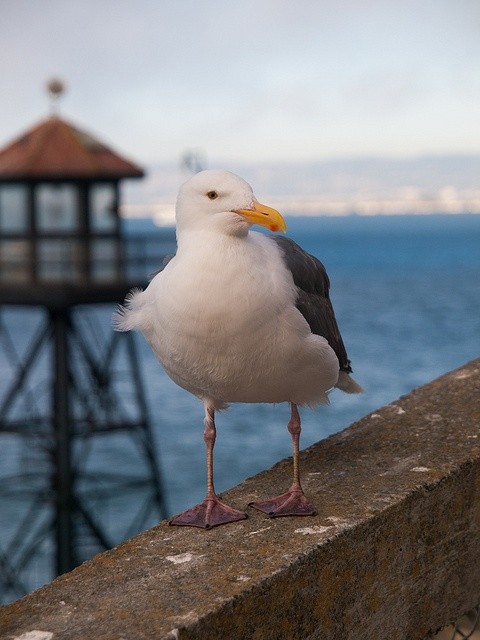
<box><xmin>113</xmin><ymin>173</ymin><xmax>364</xmax><ymax>532</ymax></box>
